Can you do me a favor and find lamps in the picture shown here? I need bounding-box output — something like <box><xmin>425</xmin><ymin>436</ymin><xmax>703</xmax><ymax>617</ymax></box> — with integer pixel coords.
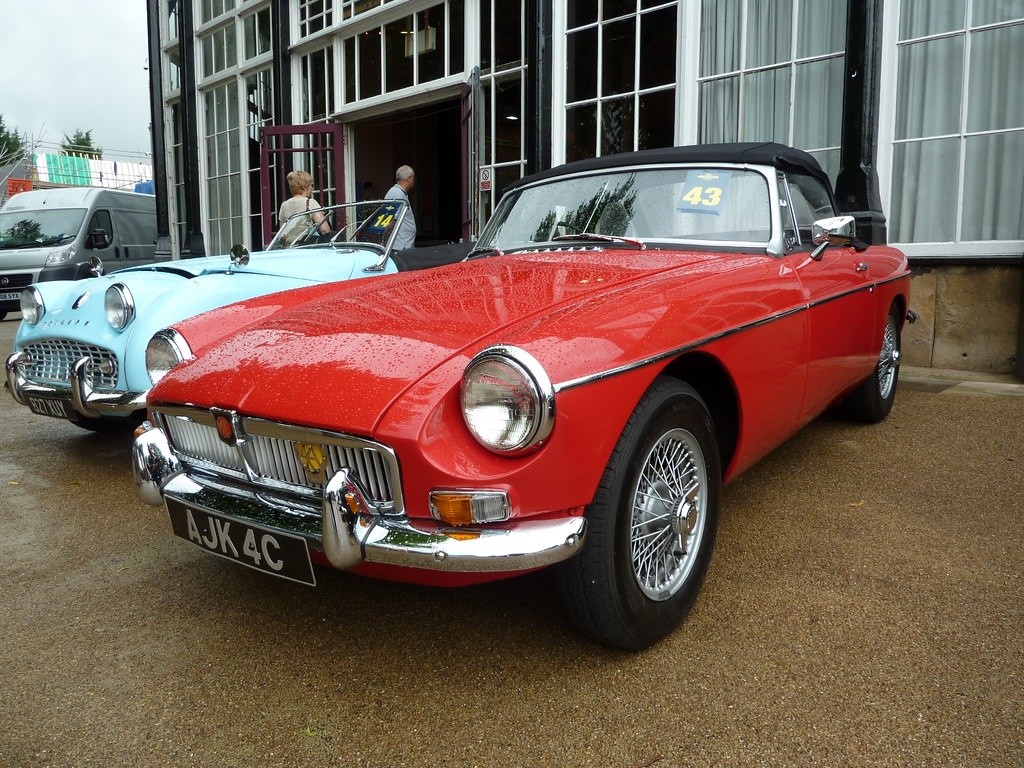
<box><xmin>504</xmin><ymin>81</ymin><xmax>520</xmax><ymax>121</ymax></box>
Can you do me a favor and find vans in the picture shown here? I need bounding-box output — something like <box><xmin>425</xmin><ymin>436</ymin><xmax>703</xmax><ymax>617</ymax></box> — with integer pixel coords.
<box><xmin>0</xmin><ymin>187</ymin><xmax>157</xmax><ymax>323</ymax></box>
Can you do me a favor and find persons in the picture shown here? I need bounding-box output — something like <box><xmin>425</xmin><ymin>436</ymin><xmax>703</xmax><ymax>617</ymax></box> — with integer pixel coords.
<box><xmin>279</xmin><ymin>169</ymin><xmax>331</xmax><ymax>246</ymax></box>
<box><xmin>384</xmin><ymin>164</ymin><xmax>416</xmax><ymax>252</ymax></box>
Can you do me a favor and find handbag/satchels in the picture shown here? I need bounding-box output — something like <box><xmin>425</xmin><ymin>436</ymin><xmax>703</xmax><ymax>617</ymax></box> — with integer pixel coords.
<box><xmin>299</xmin><ymin>196</ymin><xmax>340</xmax><ymax>245</ymax></box>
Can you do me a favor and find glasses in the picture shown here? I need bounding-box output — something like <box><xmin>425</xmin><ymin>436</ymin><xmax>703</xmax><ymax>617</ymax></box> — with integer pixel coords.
<box><xmin>309</xmin><ymin>184</ymin><xmax>314</xmax><ymax>187</ymax></box>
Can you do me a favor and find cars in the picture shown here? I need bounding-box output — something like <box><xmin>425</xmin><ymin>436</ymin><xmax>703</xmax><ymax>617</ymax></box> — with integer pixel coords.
<box><xmin>4</xmin><ymin>197</ymin><xmax>475</xmax><ymax>439</ymax></box>
<box><xmin>130</xmin><ymin>142</ymin><xmax>919</xmax><ymax>653</ymax></box>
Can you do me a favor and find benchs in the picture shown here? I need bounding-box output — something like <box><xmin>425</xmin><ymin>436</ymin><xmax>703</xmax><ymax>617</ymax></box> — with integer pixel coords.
<box><xmin>668</xmin><ymin>229</ymin><xmax>812</xmax><ymax>245</ymax></box>
<box><xmin>395</xmin><ymin>243</ymin><xmax>477</xmax><ymax>269</ymax></box>
